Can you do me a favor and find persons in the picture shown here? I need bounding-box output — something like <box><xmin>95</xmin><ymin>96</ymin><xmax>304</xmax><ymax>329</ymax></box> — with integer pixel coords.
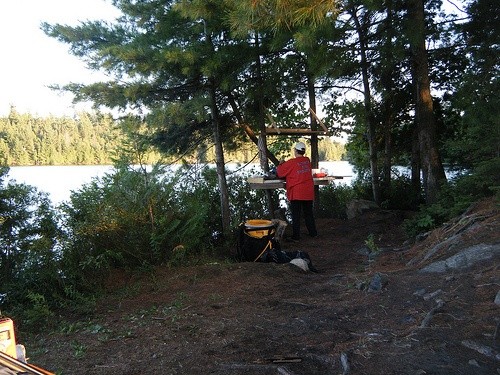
<box><xmin>276</xmin><ymin>141</ymin><xmax>318</xmax><ymax>241</ymax></box>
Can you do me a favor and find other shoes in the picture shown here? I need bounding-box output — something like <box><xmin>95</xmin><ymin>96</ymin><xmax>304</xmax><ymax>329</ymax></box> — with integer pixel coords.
<box><xmin>306</xmin><ymin>231</ymin><xmax>319</xmax><ymax>240</ymax></box>
<box><xmin>286</xmin><ymin>237</ymin><xmax>300</xmax><ymax>242</ymax></box>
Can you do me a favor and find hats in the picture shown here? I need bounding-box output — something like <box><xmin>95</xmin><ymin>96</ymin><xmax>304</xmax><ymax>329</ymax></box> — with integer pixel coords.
<box><xmin>294</xmin><ymin>142</ymin><xmax>306</xmax><ymax>151</ymax></box>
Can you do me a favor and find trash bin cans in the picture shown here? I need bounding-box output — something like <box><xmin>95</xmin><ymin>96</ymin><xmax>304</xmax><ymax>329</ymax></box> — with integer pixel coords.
<box><xmin>246</xmin><ymin>219</ymin><xmax>274</xmax><ymax>250</ymax></box>
